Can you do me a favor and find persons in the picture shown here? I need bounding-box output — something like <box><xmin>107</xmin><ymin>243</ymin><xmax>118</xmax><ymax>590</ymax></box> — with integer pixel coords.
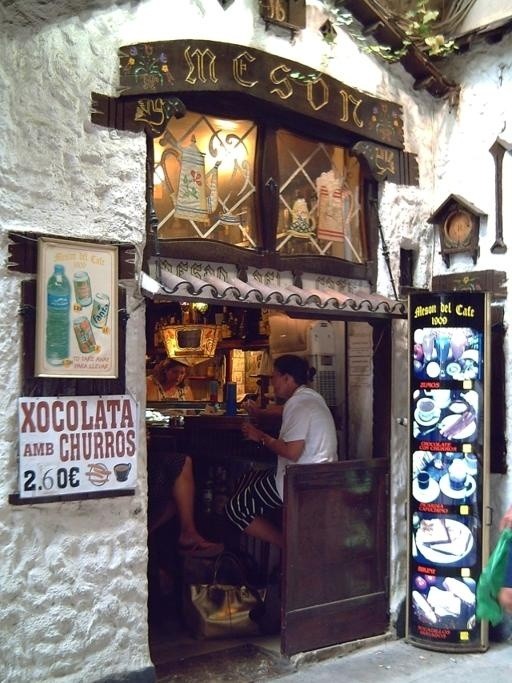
<box><xmin>147</xmin><ymin>448</ymin><xmax>225</xmax><ymax>558</ymax></box>
<box><xmin>498</xmin><ymin>504</ymin><xmax>512</xmax><ymax>612</ymax></box>
<box><xmin>146</xmin><ymin>359</ymin><xmax>193</xmax><ymax>400</ymax></box>
<box><xmin>225</xmin><ymin>352</ymin><xmax>338</xmax><ymax>553</ymax></box>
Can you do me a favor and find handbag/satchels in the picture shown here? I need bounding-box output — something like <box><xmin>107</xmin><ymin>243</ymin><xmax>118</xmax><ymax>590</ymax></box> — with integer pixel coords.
<box><xmin>185</xmin><ymin>582</ymin><xmax>272</xmax><ymax>642</ymax></box>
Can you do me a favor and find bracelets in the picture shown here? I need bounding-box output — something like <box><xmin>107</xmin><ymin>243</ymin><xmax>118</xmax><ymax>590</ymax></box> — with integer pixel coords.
<box><xmin>258</xmin><ymin>431</ymin><xmax>269</xmax><ymax>446</ymax></box>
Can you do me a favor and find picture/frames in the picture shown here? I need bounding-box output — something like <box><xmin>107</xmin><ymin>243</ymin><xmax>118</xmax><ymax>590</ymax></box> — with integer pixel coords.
<box><xmin>32</xmin><ymin>235</ymin><xmax>120</xmax><ymax>381</ymax></box>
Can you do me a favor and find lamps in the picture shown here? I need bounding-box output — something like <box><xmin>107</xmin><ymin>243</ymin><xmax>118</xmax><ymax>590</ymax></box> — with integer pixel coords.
<box><xmin>160</xmin><ymin>299</ymin><xmax>223</xmax><ymax>367</ymax></box>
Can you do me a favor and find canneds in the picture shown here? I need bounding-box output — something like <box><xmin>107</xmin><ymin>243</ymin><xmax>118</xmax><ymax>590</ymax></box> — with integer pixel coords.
<box><xmin>91</xmin><ymin>293</ymin><xmax>110</xmax><ymax>328</ymax></box>
<box><xmin>73</xmin><ymin>316</ymin><xmax>96</xmax><ymax>353</ymax></box>
<box><xmin>73</xmin><ymin>271</ymin><xmax>92</xmax><ymax>306</ymax></box>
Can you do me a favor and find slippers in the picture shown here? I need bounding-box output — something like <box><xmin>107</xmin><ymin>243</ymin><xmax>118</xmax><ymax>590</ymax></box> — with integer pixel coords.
<box><xmin>177</xmin><ymin>540</ymin><xmax>225</xmax><ymax>558</ymax></box>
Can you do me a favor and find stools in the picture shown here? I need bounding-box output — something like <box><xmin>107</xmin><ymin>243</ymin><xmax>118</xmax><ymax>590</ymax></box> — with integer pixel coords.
<box><xmin>147</xmin><ymin>403</ymin><xmax>301</xmax><ymax>642</ymax></box>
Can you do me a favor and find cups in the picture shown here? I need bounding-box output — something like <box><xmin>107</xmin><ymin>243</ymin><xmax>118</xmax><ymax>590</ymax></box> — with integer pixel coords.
<box><xmin>316</xmin><ymin>170</ymin><xmax>355</xmax><ymax>243</ymax></box>
<box><xmin>284</xmin><ymin>207</ymin><xmax>317</xmax><ymax>232</ymax></box>
<box><xmin>218</xmin><ymin>213</ymin><xmax>240</xmax><ymax>226</ymax></box>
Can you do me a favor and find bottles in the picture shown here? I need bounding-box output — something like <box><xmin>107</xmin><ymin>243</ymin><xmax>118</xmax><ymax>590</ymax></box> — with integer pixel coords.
<box><xmin>227</xmin><ymin>383</ymin><xmax>237</xmax><ymax>416</ymax></box>
<box><xmin>45</xmin><ymin>263</ymin><xmax>71</xmax><ymax>367</ymax></box>
<box><xmin>209</xmin><ymin>381</ymin><xmax>220</xmax><ymax>403</ymax></box>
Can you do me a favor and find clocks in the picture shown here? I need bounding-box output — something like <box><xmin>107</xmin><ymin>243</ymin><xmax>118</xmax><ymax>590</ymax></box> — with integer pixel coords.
<box><xmin>427</xmin><ymin>192</ymin><xmax>488</xmax><ymax>267</ymax></box>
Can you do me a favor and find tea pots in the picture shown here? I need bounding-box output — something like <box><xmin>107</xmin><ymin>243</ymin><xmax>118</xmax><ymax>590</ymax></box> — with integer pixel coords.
<box><xmin>160</xmin><ymin>134</ymin><xmax>223</xmax><ymax>226</ymax></box>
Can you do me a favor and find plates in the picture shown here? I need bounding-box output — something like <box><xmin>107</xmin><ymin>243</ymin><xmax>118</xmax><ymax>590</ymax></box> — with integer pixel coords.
<box><xmin>286</xmin><ymin>228</ymin><xmax>315</xmax><ymax>238</ymax></box>
<box><xmin>413</xmin><ymin>327</ymin><xmax>478</xmax><ymax>567</ymax></box>
